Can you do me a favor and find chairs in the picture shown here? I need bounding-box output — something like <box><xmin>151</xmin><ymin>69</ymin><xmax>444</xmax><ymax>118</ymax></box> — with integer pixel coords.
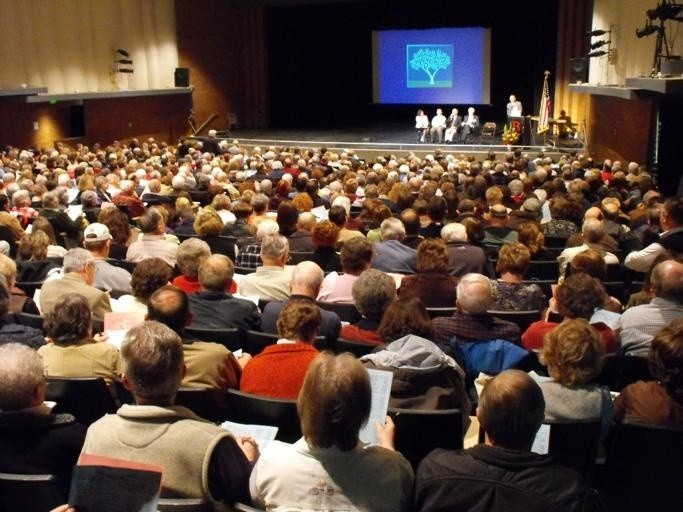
<box><xmin>480</xmin><ymin>121</ymin><xmax>496</xmax><ymax>145</ymax></box>
<box><xmin>417</xmin><ymin>115</ymin><xmax>480</xmax><ymax>145</ymax></box>
<box><xmin>1</xmin><ymin>188</ymin><xmax>681</xmax><ymax>508</ymax></box>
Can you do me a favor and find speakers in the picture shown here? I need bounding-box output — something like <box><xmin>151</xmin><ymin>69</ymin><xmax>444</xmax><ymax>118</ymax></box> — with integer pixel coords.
<box><xmin>174</xmin><ymin>68</ymin><xmax>189</xmax><ymax>87</ymax></box>
<box><xmin>570</xmin><ymin>58</ymin><xmax>588</xmax><ymax>83</ymax></box>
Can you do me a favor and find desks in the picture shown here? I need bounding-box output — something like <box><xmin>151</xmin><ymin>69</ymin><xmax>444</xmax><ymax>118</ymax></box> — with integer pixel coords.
<box><xmin>527</xmin><ymin>115</ymin><xmax>568</xmax><ymax>146</ymax></box>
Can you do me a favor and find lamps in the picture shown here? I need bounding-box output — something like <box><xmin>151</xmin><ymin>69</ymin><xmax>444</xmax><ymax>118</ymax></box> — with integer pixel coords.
<box><xmin>635</xmin><ymin>0</ymin><xmax>683</xmax><ymax>79</ymax></box>
<box><xmin>583</xmin><ymin>22</ymin><xmax>612</xmax><ymax>62</ymax></box>
<box><xmin>112</xmin><ymin>46</ymin><xmax>133</xmax><ymax>77</ymax></box>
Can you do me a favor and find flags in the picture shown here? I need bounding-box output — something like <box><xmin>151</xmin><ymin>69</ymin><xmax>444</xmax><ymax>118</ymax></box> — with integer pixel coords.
<box><xmin>536</xmin><ymin>71</ymin><xmax>549</xmax><ymax>134</ymax></box>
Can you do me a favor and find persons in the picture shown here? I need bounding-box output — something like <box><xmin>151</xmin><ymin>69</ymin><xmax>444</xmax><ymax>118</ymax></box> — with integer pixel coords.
<box><xmin>0</xmin><ymin>142</ymin><xmax>683</xmax><ymax>512</ymax></box>
<box><xmin>443</xmin><ymin>108</ymin><xmax>462</xmax><ymax>143</ymax></box>
<box><xmin>428</xmin><ymin>108</ymin><xmax>446</xmax><ymax>143</ymax></box>
<box><xmin>505</xmin><ymin>94</ymin><xmax>522</xmax><ymax>119</ymax></box>
<box><xmin>414</xmin><ymin>110</ymin><xmax>429</xmax><ymax>143</ymax></box>
<box><xmin>459</xmin><ymin>108</ymin><xmax>480</xmax><ymax>144</ymax></box>
<box><xmin>202</xmin><ymin>130</ymin><xmax>221</xmax><ymax>153</ymax></box>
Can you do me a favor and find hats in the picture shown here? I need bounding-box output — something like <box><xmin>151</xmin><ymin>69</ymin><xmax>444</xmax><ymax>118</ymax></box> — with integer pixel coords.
<box><xmin>84</xmin><ymin>223</ymin><xmax>113</xmax><ymax>243</ymax></box>
